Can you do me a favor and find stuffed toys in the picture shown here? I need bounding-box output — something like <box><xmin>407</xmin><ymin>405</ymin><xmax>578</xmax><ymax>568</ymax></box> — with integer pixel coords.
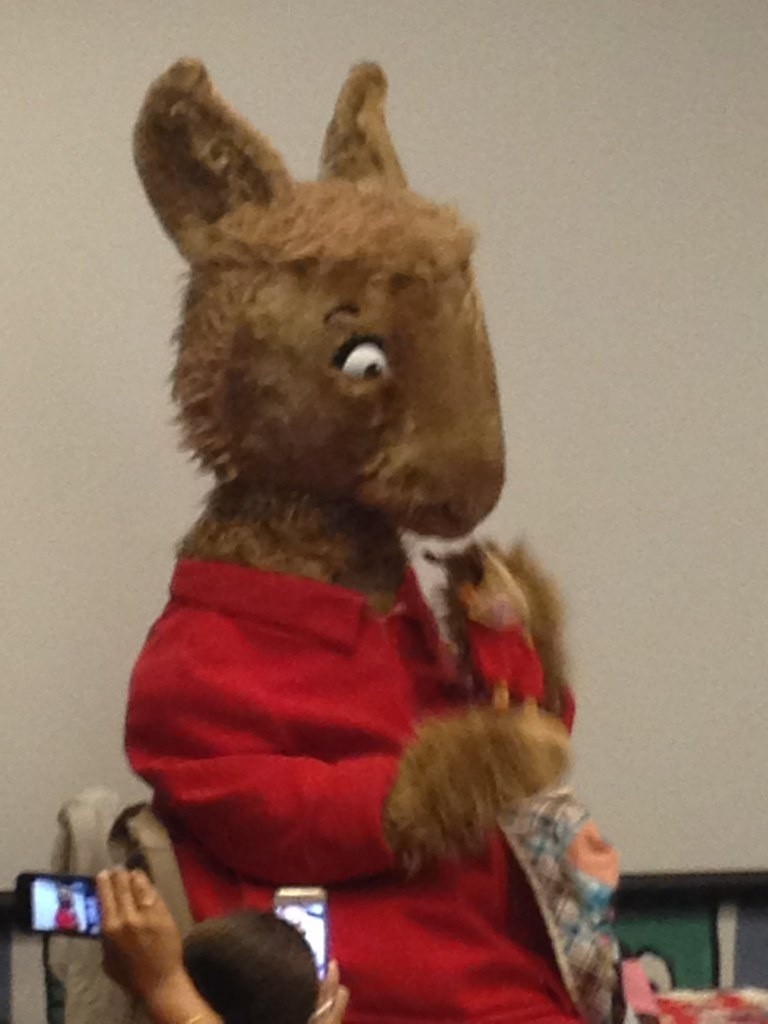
<box><xmin>124</xmin><ymin>55</ymin><xmax>695</xmax><ymax>1024</ymax></box>
<box><xmin>56</xmin><ymin>882</ymin><xmax>81</xmax><ymax>931</ymax></box>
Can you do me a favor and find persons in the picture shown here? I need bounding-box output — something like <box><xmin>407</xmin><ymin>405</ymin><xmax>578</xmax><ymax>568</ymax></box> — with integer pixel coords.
<box><xmin>97</xmin><ymin>866</ymin><xmax>350</xmax><ymax>1024</ymax></box>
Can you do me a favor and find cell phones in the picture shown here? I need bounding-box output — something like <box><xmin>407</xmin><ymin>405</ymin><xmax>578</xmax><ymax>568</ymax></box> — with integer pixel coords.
<box><xmin>14</xmin><ymin>871</ymin><xmax>103</xmax><ymax>940</ymax></box>
<box><xmin>275</xmin><ymin>886</ymin><xmax>329</xmax><ymax>983</ymax></box>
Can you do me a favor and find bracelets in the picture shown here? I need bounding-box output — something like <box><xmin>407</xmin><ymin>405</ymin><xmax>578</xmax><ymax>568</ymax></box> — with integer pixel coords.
<box><xmin>188</xmin><ymin>1014</ymin><xmax>203</xmax><ymax>1024</ymax></box>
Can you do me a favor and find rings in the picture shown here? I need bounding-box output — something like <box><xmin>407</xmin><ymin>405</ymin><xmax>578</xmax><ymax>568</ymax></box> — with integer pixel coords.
<box><xmin>140</xmin><ymin>894</ymin><xmax>155</xmax><ymax>908</ymax></box>
